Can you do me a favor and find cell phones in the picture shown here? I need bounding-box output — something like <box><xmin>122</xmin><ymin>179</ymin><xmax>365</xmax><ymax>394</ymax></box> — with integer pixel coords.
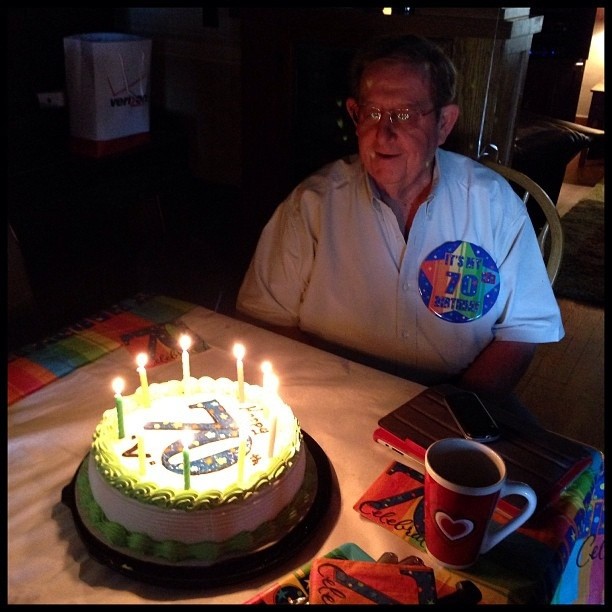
<box><xmin>443</xmin><ymin>392</ymin><xmax>501</xmax><ymax>443</ymax></box>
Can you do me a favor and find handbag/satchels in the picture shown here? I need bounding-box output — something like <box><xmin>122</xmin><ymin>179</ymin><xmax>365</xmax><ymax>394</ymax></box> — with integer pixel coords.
<box><xmin>63</xmin><ymin>32</ymin><xmax>152</xmax><ymax>141</ymax></box>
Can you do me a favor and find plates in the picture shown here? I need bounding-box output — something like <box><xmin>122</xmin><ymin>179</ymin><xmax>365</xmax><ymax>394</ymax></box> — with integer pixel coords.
<box><xmin>236</xmin><ymin>543</ymin><xmax>379</xmax><ymax>604</ymax></box>
<box><xmin>62</xmin><ymin>428</ymin><xmax>331</xmax><ymax>588</ymax></box>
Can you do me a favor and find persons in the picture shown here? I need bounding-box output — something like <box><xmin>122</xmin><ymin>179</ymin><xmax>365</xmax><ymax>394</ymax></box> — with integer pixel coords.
<box><xmin>236</xmin><ymin>34</ymin><xmax>567</xmax><ymax>393</ymax></box>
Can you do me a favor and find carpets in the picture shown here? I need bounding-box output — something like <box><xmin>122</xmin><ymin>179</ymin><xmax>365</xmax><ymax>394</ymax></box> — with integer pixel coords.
<box><xmin>541</xmin><ymin>172</ymin><xmax>607</xmax><ymax>313</ymax></box>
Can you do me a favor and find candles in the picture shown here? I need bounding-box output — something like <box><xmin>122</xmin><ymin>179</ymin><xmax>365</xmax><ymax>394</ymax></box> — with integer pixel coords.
<box><xmin>114</xmin><ymin>393</ymin><xmax>124</xmax><ymax>440</ymax></box>
<box><xmin>136</xmin><ymin>367</ymin><xmax>151</xmax><ymax>408</ymax></box>
<box><xmin>262</xmin><ymin>375</ymin><xmax>270</xmax><ymax>419</ymax></box>
<box><xmin>137</xmin><ymin>433</ymin><xmax>145</xmax><ymax>475</ymax></box>
<box><xmin>236</xmin><ymin>359</ymin><xmax>245</xmax><ymax>401</ymax></box>
<box><xmin>268</xmin><ymin>416</ymin><xmax>277</xmax><ymax>457</ymax></box>
<box><xmin>238</xmin><ymin>438</ymin><xmax>246</xmax><ymax>482</ymax></box>
<box><xmin>181</xmin><ymin>350</ymin><xmax>191</xmax><ymax>395</ymax></box>
<box><xmin>182</xmin><ymin>448</ymin><xmax>190</xmax><ymax>490</ymax></box>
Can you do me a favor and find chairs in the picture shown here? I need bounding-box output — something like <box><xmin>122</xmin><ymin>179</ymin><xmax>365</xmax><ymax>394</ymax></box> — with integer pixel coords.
<box><xmin>481</xmin><ymin>158</ymin><xmax>564</xmax><ymax>288</ymax></box>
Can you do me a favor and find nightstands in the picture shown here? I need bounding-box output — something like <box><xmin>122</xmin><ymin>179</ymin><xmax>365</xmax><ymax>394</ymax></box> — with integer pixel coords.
<box><xmin>588</xmin><ymin>79</ymin><xmax>605</xmax><ymax>125</ymax></box>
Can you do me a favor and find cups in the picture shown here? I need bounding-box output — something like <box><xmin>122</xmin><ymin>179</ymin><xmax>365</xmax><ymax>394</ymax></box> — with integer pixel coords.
<box><xmin>422</xmin><ymin>440</ymin><xmax>537</xmax><ymax>569</ymax></box>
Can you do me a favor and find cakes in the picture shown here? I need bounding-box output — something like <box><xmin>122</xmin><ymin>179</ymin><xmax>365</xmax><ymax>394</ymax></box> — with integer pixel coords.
<box><xmin>72</xmin><ymin>376</ymin><xmax>319</xmax><ymax>566</ymax></box>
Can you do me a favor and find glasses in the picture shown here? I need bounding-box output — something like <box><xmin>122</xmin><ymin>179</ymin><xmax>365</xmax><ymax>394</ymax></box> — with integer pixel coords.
<box><xmin>350</xmin><ymin>102</ymin><xmax>440</xmax><ymax>126</ymax></box>
